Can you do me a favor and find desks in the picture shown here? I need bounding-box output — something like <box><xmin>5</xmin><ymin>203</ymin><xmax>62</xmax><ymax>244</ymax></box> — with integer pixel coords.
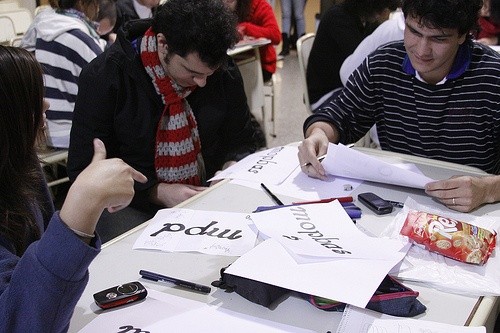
<box><xmin>227</xmin><ymin>38</ymin><xmax>271</xmax><ymax>128</ymax></box>
<box><xmin>67</xmin><ymin>142</ymin><xmax>500</xmax><ymax>333</ymax></box>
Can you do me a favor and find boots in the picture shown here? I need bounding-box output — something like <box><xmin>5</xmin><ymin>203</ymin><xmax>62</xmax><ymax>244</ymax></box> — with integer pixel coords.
<box><xmin>278</xmin><ymin>32</ymin><xmax>289</xmax><ymax>56</ymax></box>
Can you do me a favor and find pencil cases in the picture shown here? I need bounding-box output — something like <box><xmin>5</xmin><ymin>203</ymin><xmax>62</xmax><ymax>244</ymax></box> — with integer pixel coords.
<box><xmin>300</xmin><ymin>274</ymin><xmax>426</xmax><ymax>317</ymax></box>
<box><xmin>211</xmin><ymin>263</ymin><xmax>291</xmax><ymax>307</ymax></box>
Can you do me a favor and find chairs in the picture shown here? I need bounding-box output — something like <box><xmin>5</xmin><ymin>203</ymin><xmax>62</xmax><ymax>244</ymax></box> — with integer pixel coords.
<box><xmin>263</xmin><ymin>41</ymin><xmax>283</xmax><ymax>137</ymax></box>
<box><xmin>296</xmin><ymin>33</ymin><xmax>316</xmax><ymax>115</ymax></box>
<box><xmin>37</xmin><ymin>150</ymin><xmax>69</xmax><ymax>187</ymax></box>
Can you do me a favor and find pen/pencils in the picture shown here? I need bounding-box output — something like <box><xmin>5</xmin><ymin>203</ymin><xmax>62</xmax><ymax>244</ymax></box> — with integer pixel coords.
<box><xmin>301</xmin><ymin>144</ymin><xmax>354</xmax><ymax>167</ymax></box>
<box><xmin>292</xmin><ymin>197</ymin><xmax>353</xmax><ymax>205</ymax></box>
<box><xmin>465</xmin><ymin>296</ymin><xmax>484</xmax><ymax>326</ymax></box>
<box><xmin>261</xmin><ymin>183</ymin><xmax>283</xmax><ymax>205</ymax></box>
<box><xmin>140</xmin><ymin>270</ymin><xmax>211</xmax><ymax>293</ymax></box>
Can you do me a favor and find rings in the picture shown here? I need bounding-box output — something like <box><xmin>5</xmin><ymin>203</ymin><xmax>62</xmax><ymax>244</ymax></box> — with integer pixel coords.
<box><xmin>452</xmin><ymin>199</ymin><xmax>455</xmax><ymax>204</ymax></box>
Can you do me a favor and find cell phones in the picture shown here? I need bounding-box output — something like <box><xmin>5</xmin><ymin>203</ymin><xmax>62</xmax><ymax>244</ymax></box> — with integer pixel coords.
<box><xmin>357</xmin><ymin>192</ymin><xmax>393</xmax><ymax>214</ymax></box>
<box><xmin>93</xmin><ymin>281</ymin><xmax>147</xmax><ymax>309</ymax></box>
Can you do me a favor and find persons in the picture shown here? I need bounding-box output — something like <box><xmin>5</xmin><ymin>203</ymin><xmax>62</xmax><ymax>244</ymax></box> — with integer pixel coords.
<box><xmin>298</xmin><ymin>0</ymin><xmax>500</xmax><ymax>212</ymax></box>
<box><xmin>225</xmin><ymin>0</ymin><xmax>282</xmax><ymax>83</ymax></box>
<box><xmin>306</xmin><ymin>0</ymin><xmax>397</xmax><ymax>113</ymax></box>
<box><xmin>96</xmin><ymin>0</ymin><xmax>138</xmax><ymax>43</ymax></box>
<box><xmin>280</xmin><ymin>0</ymin><xmax>307</xmax><ymax>56</ymax></box>
<box><xmin>340</xmin><ymin>0</ymin><xmax>404</xmax><ymax>143</ymax></box>
<box><xmin>470</xmin><ymin>0</ymin><xmax>500</xmax><ymax>46</ymax></box>
<box><xmin>125</xmin><ymin>0</ymin><xmax>160</xmax><ymax>20</ymax></box>
<box><xmin>23</xmin><ymin>0</ymin><xmax>59</xmax><ymax>53</ymax></box>
<box><xmin>0</xmin><ymin>44</ymin><xmax>147</xmax><ymax>333</ymax></box>
<box><xmin>35</xmin><ymin>0</ymin><xmax>113</xmax><ymax>150</ymax></box>
<box><xmin>67</xmin><ymin>0</ymin><xmax>259</xmax><ymax>213</ymax></box>
